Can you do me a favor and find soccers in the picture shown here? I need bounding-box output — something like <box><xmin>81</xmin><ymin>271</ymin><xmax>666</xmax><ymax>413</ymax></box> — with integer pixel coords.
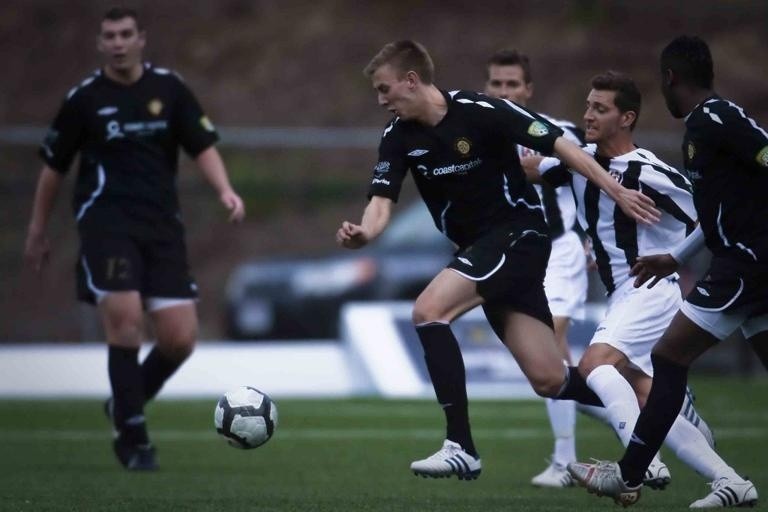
<box><xmin>214</xmin><ymin>386</ymin><xmax>279</xmax><ymax>449</ymax></box>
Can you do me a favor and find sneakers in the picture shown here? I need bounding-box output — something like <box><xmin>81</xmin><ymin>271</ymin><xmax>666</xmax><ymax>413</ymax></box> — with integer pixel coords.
<box><xmin>566</xmin><ymin>460</ymin><xmax>644</xmax><ymax>508</ymax></box>
<box><xmin>688</xmin><ymin>474</ymin><xmax>760</xmax><ymax>510</ymax></box>
<box><xmin>637</xmin><ymin>459</ymin><xmax>673</xmax><ymax>492</ymax></box>
<box><xmin>111</xmin><ymin>415</ymin><xmax>159</xmax><ymax>474</ymax></box>
<box><xmin>530</xmin><ymin>457</ymin><xmax>581</xmax><ymax>488</ymax></box>
<box><xmin>677</xmin><ymin>386</ymin><xmax>715</xmax><ymax>453</ymax></box>
<box><xmin>408</xmin><ymin>439</ymin><xmax>483</xmax><ymax>482</ymax></box>
<box><xmin>104</xmin><ymin>397</ymin><xmax>122</xmax><ymax>438</ymax></box>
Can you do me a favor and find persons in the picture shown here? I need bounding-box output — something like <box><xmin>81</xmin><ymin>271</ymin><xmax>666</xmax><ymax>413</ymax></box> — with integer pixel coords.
<box><xmin>517</xmin><ymin>70</ymin><xmax>760</xmax><ymax>510</ymax></box>
<box><xmin>22</xmin><ymin>7</ymin><xmax>245</xmax><ymax>472</ymax></box>
<box><xmin>336</xmin><ymin>36</ymin><xmax>715</xmax><ymax>479</ymax></box>
<box><xmin>565</xmin><ymin>29</ymin><xmax>765</xmax><ymax>508</ymax></box>
<box><xmin>481</xmin><ymin>48</ymin><xmax>614</xmax><ymax>489</ymax></box>
